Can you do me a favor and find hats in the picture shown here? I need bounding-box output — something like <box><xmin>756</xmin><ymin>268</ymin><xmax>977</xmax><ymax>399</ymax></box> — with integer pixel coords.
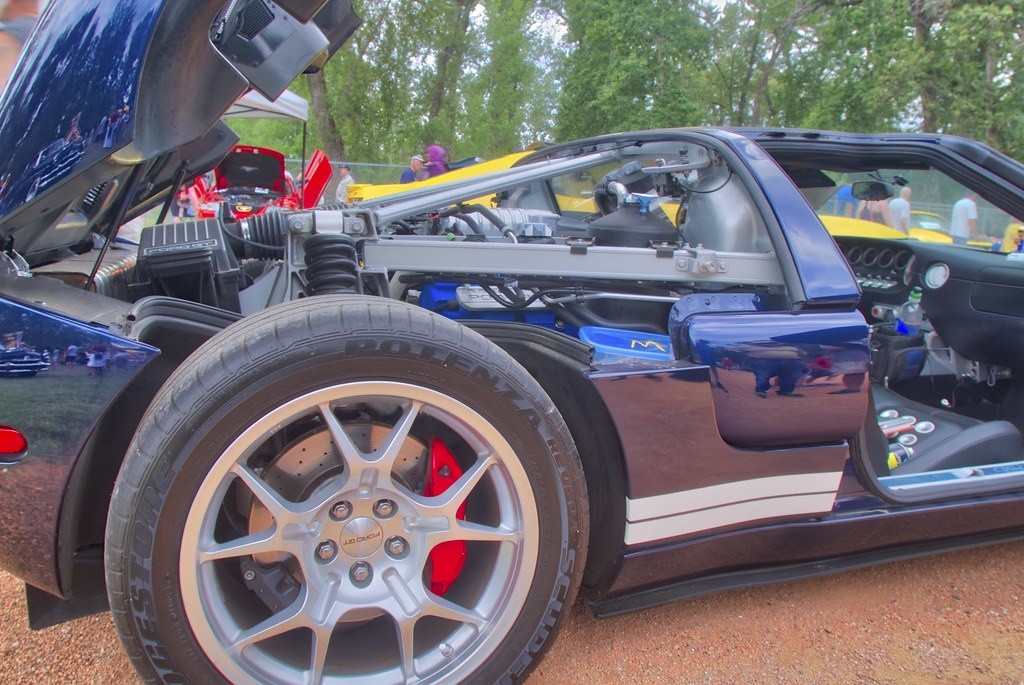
<box><xmin>339</xmin><ymin>164</ymin><xmax>349</xmax><ymax>170</ymax></box>
<box><xmin>411</xmin><ymin>155</ymin><xmax>425</xmax><ymax>162</ymax></box>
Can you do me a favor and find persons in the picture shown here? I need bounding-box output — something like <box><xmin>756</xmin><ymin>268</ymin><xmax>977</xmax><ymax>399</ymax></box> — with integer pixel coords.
<box><xmin>998</xmin><ymin>215</ymin><xmax>1024</xmax><ymax>252</ymax></box>
<box><xmin>335</xmin><ymin>164</ymin><xmax>354</xmax><ymax>203</ymax></box>
<box><xmin>425</xmin><ymin>144</ymin><xmax>449</xmax><ymax>177</ymax></box>
<box><xmin>42</xmin><ymin>342</ymin><xmax>124</xmax><ymax>379</ymax></box>
<box><xmin>400</xmin><ymin>154</ymin><xmax>426</xmax><ymax>184</ymax></box>
<box><xmin>696</xmin><ymin>338</ymin><xmax>869</xmax><ymax>397</ymax></box>
<box><xmin>0</xmin><ymin>331</ymin><xmax>37</xmax><ymax>350</ymax></box>
<box><xmin>833</xmin><ymin>177</ymin><xmax>911</xmax><ymax>237</ymax></box>
<box><xmin>949</xmin><ymin>189</ymin><xmax>982</xmax><ymax>244</ymax></box>
<box><xmin>92</xmin><ymin>105</ymin><xmax>131</xmax><ymax>151</ymax></box>
<box><xmin>0</xmin><ymin>171</ymin><xmax>14</xmax><ymax>210</ymax></box>
<box><xmin>65</xmin><ymin>115</ymin><xmax>84</xmax><ymax>142</ymax></box>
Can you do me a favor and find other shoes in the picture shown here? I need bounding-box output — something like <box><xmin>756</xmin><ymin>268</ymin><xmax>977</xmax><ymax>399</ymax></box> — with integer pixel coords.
<box><xmin>779</xmin><ymin>391</ymin><xmax>802</xmax><ymax>397</ymax></box>
<box><xmin>757</xmin><ymin>391</ymin><xmax>767</xmax><ymax>396</ymax></box>
<box><xmin>715</xmin><ymin>382</ymin><xmax>728</xmax><ymax>392</ymax></box>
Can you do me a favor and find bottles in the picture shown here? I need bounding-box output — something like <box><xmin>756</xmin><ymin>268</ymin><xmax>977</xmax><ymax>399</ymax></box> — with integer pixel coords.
<box><xmin>443</xmin><ymin>207</ymin><xmax>553</xmax><ymax>238</ymax></box>
<box><xmin>894</xmin><ymin>286</ymin><xmax>923</xmax><ymax>336</ymax></box>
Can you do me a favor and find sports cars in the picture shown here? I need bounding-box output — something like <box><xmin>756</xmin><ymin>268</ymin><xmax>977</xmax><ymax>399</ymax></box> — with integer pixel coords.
<box><xmin>0</xmin><ymin>0</ymin><xmax>1023</xmax><ymax>684</ymax></box>
